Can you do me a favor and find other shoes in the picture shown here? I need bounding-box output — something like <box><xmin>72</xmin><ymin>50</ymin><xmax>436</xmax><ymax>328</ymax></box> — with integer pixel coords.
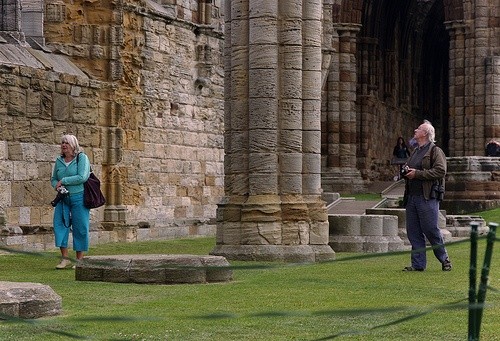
<box><xmin>402</xmin><ymin>266</ymin><xmax>424</xmax><ymax>272</ymax></box>
<box><xmin>55</xmin><ymin>259</ymin><xmax>71</xmax><ymax>269</ymax></box>
<box><xmin>442</xmin><ymin>261</ymin><xmax>452</xmax><ymax>272</ymax></box>
<box><xmin>72</xmin><ymin>263</ymin><xmax>78</xmax><ymax>270</ymax></box>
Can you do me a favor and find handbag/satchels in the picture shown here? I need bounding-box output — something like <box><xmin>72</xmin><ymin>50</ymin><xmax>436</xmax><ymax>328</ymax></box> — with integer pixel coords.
<box><xmin>76</xmin><ymin>153</ymin><xmax>105</xmax><ymax>209</ymax></box>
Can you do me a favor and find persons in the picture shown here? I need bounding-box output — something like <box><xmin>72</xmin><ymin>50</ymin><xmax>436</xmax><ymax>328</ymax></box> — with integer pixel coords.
<box><xmin>50</xmin><ymin>135</ymin><xmax>104</xmax><ymax>270</ymax></box>
<box><xmin>399</xmin><ymin>119</ymin><xmax>454</xmax><ymax>272</ymax></box>
<box><xmin>393</xmin><ymin>136</ymin><xmax>410</xmax><ymax>181</ymax></box>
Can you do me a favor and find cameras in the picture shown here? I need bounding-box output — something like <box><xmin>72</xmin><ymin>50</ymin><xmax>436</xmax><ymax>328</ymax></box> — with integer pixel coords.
<box><xmin>59</xmin><ymin>186</ymin><xmax>68</xmax><ymax>194</ymax></box>
<box><xmin>404</xmin><ymin>166</ymin><xmax>409</xmax><ymax>173</ymax></box>
